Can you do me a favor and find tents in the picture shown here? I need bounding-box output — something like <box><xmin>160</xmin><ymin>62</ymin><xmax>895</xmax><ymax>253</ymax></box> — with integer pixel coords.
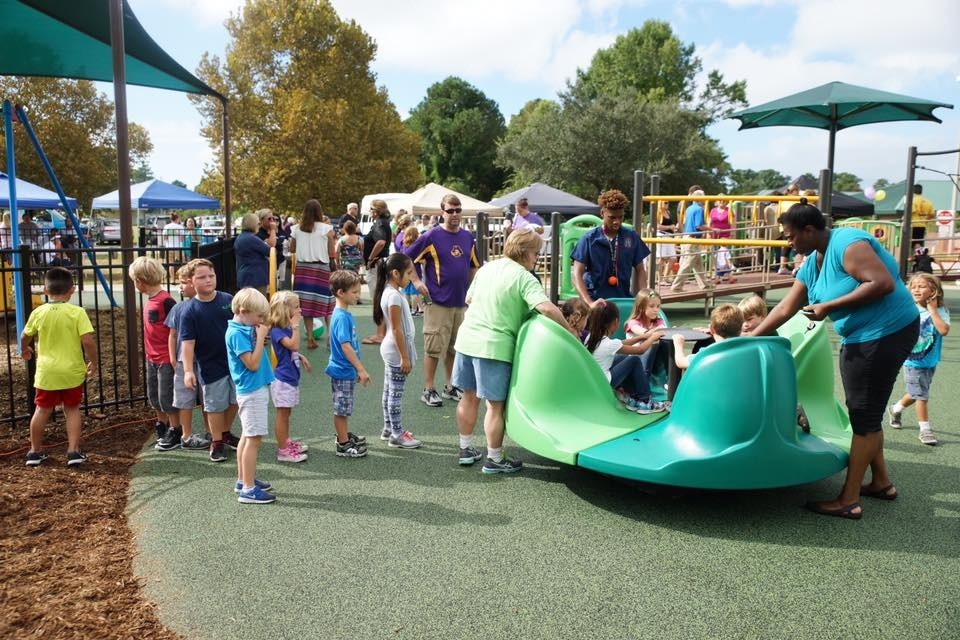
<box><xmin>758</xmin><ymin>175</ymin><xmax>874</xmax><ymax>220</ymax></box>
<box><xmin>360</xmin><ymin>180</ymin><xmax>503</xmax><ymax>260</ymax></box>
<box><xmin>485</xmin><ymin>183</ymin><xmax>600</xmax><ymax>215</ymax></box>
<box><xmin>88</xmin><ymin>179</ymin><xmax>223</xmax><ymax>240</ymax></box>
<box><xmin>0</xmin><ymin>171</ymin><xmax>79</xmax><ymax>218</ymax></box>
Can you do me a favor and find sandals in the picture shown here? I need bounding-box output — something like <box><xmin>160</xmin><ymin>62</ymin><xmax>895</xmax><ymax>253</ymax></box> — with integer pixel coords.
<box><xmin>859</xmin><ymin>482</ymin><xmax>898</xmax><ymax>502</ymax></box>
<box><xmin>814</xmin><ymin>500</ymin><xmax>863</xmax><ymax>520</ymax></box>
<box><xmin>797</xmin><ymin>402</ymin><xmax>810</xmax><ymax>433</ymax></box>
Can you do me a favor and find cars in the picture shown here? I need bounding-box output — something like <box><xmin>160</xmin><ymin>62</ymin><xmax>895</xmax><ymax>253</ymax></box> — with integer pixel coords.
<box><xmin>96</xmin><ymin>219</ymin><xmax>120</xmax><ymax>244</ymax></box>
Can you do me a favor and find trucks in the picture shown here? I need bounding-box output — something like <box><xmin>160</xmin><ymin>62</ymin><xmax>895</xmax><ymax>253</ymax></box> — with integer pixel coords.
<box><xmin>19</xmin><ymin>209</ymin><xmax>89</xmax><ymax>243</ymax></box>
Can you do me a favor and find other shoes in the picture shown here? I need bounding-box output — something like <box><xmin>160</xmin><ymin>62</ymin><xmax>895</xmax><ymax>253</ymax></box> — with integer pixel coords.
<box><xmin>778</xmin><ymin>267</ymin><xmax>788</xmax><ymax>274</ymax></box>
<box><xmin>307</xmin><ymin>339</ymin><xmax>318</xmax><ymax>349</ymax></box>
<box><xmin>412</xmin><ymin>311</ymin><xmax>423</xmax><ymax>317</ymax></box>
<box><xmin>656</xmin><ymin>272</ymin><xmax>736</xmax><ymax>293</ymax></box>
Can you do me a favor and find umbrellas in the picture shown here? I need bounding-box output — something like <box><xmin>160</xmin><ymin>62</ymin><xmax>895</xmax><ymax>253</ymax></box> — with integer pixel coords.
<box><xmin>727</xmin><ymin>80</ymin><xmax>954</xmax><ymax>216</ymax></box>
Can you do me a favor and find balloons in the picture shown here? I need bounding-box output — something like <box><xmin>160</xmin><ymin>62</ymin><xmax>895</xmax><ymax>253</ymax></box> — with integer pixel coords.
<box><xmin>865</xmin><ymin>188</ymin><xmax>886</xmax><ymax>200</ymax></box>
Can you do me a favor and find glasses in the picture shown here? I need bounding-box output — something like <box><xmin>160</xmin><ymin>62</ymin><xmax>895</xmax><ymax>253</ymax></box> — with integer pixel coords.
<box><xmin>370</xmin><ymin>207</ymin><xmax>376</xmax><ymax>211</ymax></box>
<box><xmin>443</xmin><ymin>208</ymin><xmax>461</xmax><ymax>214</ymax></box>
<box><xmin>264</xmin><ymin>217</ymin><xmax>274</xmax><ymax>221</ymax></box>
<box><xmin>274</xmin><ymin>218</ymin><xmax>280</xmax><ymax>222</ymax></box>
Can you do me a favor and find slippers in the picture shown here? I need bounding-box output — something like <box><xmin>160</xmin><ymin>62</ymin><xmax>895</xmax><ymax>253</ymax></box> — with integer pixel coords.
<box><xmin>362</xmin><ymin>336</ymin><xmax>384</xmax><ymax>345</ymax></box>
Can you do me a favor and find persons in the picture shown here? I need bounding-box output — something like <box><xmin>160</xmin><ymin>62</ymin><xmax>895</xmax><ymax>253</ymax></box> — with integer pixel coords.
<box><xmin>764</xmin><ymin>184</ymin><xmax>816</xmax><ymax>274</ymax></box>
<box><xmin>20</xmin><ymin>267</ymin><xmax>97</xmax><ymax>464</ymax></box>
<box><xmin>664</xmin><ymin>296</ymin><xmax>810</xmax><ymax>433</ymax></box>
<box><xmin>129</xmin><ymin>195</ymin><xmax>576</xmax><ymax>503</ymax></box>
<box><xmin>656</xmin><ymin>186</ymin><xmax>736</xmax><ymax>292</ymax></box>
<box><xmin>749</xmin><ymin>198</ymin><xmax>920</xmax><ymax>518</ymax></box>
<box><xmin>571</xmin><ymin>190</ymin><xmax>651</xmax><ymax>307</ymax></box>
<box><xmin>562</xmin><ymin>287</ymin><xmax>676</xmax><ymax>414</ymax></box>
<box><xmin>0</xmin><ymin>212</ymin><xmax>80</xmax><ymax>267</ymax></box>
<box><xmin>908</xmin><ymin>248</ymin><xmax>945</xmax><ymax>274</ymax></box>
<box><xmin>911</xmin><ymin>184</ymin><xmax>935</xmax><ymax>248</ymax></box>
<box><xmin>888</xmin><ymin>271</ymin><xmax>951</xmax><ymax>443</ymax></box>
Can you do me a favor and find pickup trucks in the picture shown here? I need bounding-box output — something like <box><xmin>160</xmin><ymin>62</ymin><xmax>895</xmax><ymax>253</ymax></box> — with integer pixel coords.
<box><xmin>146</xmin><ymin>215</ymin><xmax>244</xmax><ymax>244</ymax></box>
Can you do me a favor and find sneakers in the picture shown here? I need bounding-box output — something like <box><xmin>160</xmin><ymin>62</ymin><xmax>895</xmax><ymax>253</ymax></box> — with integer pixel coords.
<box><xmin>482</xmin><ymin>450</ymin><xmax>523</xmax><ymax>474</ymax></box>
<box><xmin>237</xmin><ymin>487</ymin><xmax>276</xmax><ymax>504</ymax></box>
<box><xmin>380</xmin><ymin>428</ymin><xmax>414</xmax><ymax>441</ymax></box>
<box><xmin>887</xmin><ymin>405</ymin><xmax>902</xmax><ymax>428</ymax></box>
<box><xmin>154</xmin><ymin>429</ymin><xmax>369</xmax><ymax>462</ymax></box>
<box><xmin>442</xmin><ymin>383</ymin><xmax>464</xmax><ymax>401</ymax></box>
<box><xmin>617</xmin><ymin>384</ymin><xmax>672</xmax><ymax>414</ymax></box>
<box><xmin>26</xmin><ymin>451</ymin><xmax>51</xmax><ymax>465</ymax></box>
<box><xmin>919</xmin><ymin>428</ymin><xmax>938</xmax><ymax>444</ymax></box>
<box><xmin>421</xmin><ymin>388</ymin><xmax>442</xmax><ymax>406</ymax></box>
<box><xmin>234</xmin><ymin>479</ymin><xmax>271</xmax><ymax>494</ymax></box>
<box><xmin>458</xmin><ymin>446</ymin><xmax>482</xmax><ymax>465</ymax></box>
<box><xmin>388</xmin><ymin>430</ymin><xmax>421</xmax><ymax>449</ymax></box>
<box><xmin>67</xmin><ymin>451</ymin><xmax>88</xmax><ymax>465</ymax></box>
